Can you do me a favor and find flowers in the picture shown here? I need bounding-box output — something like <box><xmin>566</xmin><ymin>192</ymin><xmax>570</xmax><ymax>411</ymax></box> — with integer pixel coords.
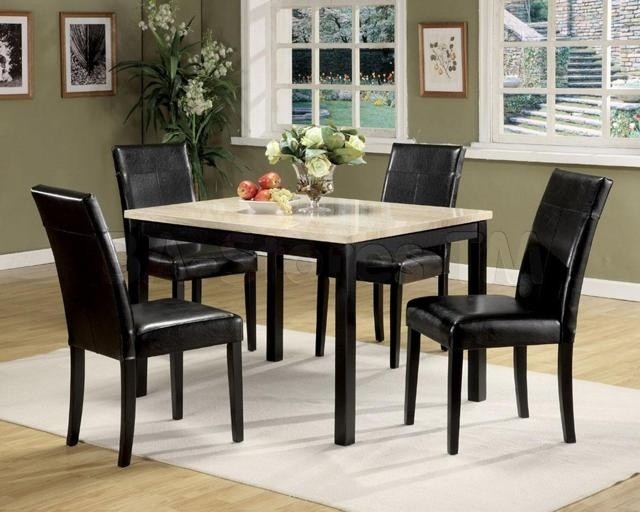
<box><xmin>106</xmin><ymin>1</ymin><xmax>250</xmax><ymax>206</ymax></box>
<box><xmin>264</xmin><ymin>121</ymin><xmax>369</xmax><ymax>176</ymax></box>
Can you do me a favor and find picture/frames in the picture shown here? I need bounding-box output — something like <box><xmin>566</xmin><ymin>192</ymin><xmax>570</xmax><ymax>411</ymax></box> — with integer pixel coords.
<box><xmin>0</xmin><ymin>8</ymin><xmax>35</xmax><ymax>101</ymax></box>
<box><xmin>58</xmin><ymin>8</ymin><xmax>118</xmax><ymax>103</ymax></box>
<box><xmin>414</xmin><ymin>19</ymin><xmax>471</xmax><ymax>101</ymax></box>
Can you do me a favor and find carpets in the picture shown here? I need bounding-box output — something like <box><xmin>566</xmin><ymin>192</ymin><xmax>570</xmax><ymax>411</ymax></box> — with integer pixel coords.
<box><xmin>0</xmin><ymin>324</ymin><xmax>639</xmax><ymax>512</ymax></box>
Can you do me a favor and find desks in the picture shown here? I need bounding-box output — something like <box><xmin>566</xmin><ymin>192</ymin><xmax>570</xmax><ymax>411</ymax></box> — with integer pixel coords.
<box><xmin>123</xmin><ymin>188</ymin><xmax>494</xmax><ymax>452</ymax></box>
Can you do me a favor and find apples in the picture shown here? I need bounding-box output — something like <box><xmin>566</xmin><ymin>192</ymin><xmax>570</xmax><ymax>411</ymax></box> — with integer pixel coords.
<box><xmin>237</xmin><ymin>171</ymin><xmax>281</xmax><ymax>201</ymax></box>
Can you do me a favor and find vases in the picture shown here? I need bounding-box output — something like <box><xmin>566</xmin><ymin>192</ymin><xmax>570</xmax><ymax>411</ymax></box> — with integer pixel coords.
<box><xmin>293</xmin><ymin>158</ymin><xmax>344</xmax><ymax>220</ymax></box>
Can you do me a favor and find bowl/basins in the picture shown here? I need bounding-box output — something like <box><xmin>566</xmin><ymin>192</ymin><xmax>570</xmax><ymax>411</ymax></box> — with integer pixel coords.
<box><xmin>241</xmin><ymin>196</ymin><xmax>302</xmax><ymax>213</ymax></box>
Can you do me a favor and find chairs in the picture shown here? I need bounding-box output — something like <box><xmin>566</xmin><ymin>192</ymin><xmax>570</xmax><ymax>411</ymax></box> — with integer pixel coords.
<box><xmin>28</xmin><ymin>181</ymin><xmax>247</xmax><ymax>471</ymax></box>
<box><xmin>315</xmin><ymin>142</ymin><xmax>467</xmax><ymax>370</ymax></box>
<box><xmin>401</xmin><ymin>162</ymin><xmax>617</xmax><ymax>458</ymax></box>
<box><xmin>109</xmin><ymin>141</ymin><xmax>260</xmax><ymax>354</ymax></box>
